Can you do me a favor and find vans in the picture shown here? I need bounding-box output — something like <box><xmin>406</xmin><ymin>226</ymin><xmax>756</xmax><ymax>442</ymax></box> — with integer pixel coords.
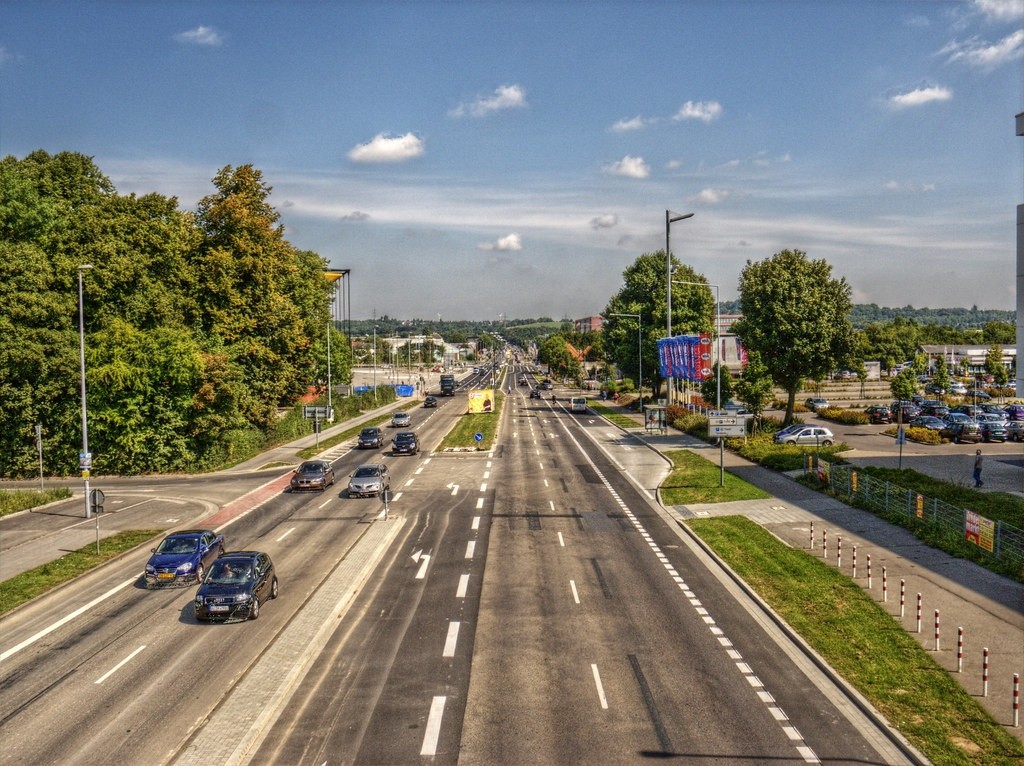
<box><xmin>569</xmin><ymin>396</ymin><xmax>588</xmax><ymax>413</ymax></box>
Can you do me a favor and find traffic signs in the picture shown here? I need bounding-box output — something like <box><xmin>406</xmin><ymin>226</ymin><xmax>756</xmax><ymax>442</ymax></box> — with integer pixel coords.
<box><xmin>708</xmin><ymin>410</ymin><xmax>747</xmax><ymax>438</ymax></box>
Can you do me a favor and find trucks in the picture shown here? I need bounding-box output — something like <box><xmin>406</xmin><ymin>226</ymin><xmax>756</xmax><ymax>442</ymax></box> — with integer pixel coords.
<box><xmin>440</xmin><ymin>375</ymin><xmax>457</xmax><ymax>398</ymax></box>
<box><xmin>473</xmin><ymin>365</ymin><xmax>488</xmax><ymax>376</ymax></box>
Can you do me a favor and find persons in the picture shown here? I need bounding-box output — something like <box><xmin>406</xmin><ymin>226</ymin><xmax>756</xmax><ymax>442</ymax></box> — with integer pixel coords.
<box><xmin>370</xmin><ymin>469</ymin><xmax>379</xmax><ymax>476</ymax></box>
<box><xmin>218</xmin><ymin>564</ymin><xmax>236</xmax><ymax>578</ymax></box>
<box><xmin>973</xmin><ymin>449</ymin><xmax>984</xmax><ymax>488</ymax></box>
<box><xmin>508</xmin><ymin>383</ymin><xmax>511</xmax><ymax>394</ymax></box>
<box><xmin>602</xmin><ymin>389</ymin><xmax>606</xmax><ymax>402</ymax></box>
<box><xmin>614</xmin><ymin>391</ymin><xmax>619</xmax><ymax>403</ymax></box>
<box><xmin>173</xmin><ymin>539</ymin><xmax>203</xmax><ymax>550</ymax></box>
<box><xmin>302</xmin><ymin>466</ymin><xmax>323</xmax><ymax>473</ymax></box>
<box><xmin>552</xmin><ymin>394</ymin><xmax>556</xmax><ymax>403</ymax></box>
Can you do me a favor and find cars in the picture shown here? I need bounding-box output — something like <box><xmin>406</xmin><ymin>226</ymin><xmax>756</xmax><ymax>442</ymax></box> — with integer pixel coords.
<box><xmin>392</xmin><ymin>412</ymin><xmax>411</xmax><ymax>428</ymax></box>
<box><xmin>805</xmin><ymin>397</ymin><xmax>831</xmax><ymax>412</ymax></box>
<box><xmin>348</xmin><ymin>464</ymin><xmax>393</xmax><ymax>498</ymax></box>
<box><xmin>424</xmin><ymin>396</ymin><xmax>438</xmax><ymax>407</ymax></box>
<box><xmin>144</xmin><ymin>530</ymin><xmax>225</xmax><ymax>589</ymax></box>
<box><xmin>865</xmin><ymin>374</ymin><xmax>1024</xmax><ymax>444</ymax></box>
<box><xmin>195</xmin><ymin>551</ymin><xmax>279</xmax><ymax>623</ymax></box>
<box><xmin>290</xmin><ymin>460</ymin><xmax>338</xmax><ymax>494</ymax></box>
<box><xmin>358</xmin><ymin>427</ymin><xmax>420</xmax><ymax>456</ymax></box>
<box><xmin>773</xmin><ymin>424</ymin><xmax>837</xmax><ymax>448</ymax></box>
<box><xmin>519</xmin><ymin>364</ymin><xmax>604</xmax><ymax>400</ymax></box>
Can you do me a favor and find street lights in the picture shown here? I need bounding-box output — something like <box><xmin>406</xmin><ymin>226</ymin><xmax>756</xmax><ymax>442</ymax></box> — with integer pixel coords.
<box><xmin>372</xmin><ymin>324</ymin><xmax>436</xmax><ymax>403</ymax></box>
<box><xmin>78</xmin><ymin>264</ymin><xmax>96</xmax><ymax>518</ymax></box>
<box><xmin>666</xmin><ymin>210</ymin><xmax>695</xmax><ymax>405</ymax></box>
<box><xmin>672</xmin><ymin>279</ymin><xmax>728</xmax><ymax>488</ymax></box>
<box><xmin>609</xmin><ymin>312</ymin><xmax>644</xmax><ymax>414</ymax></box>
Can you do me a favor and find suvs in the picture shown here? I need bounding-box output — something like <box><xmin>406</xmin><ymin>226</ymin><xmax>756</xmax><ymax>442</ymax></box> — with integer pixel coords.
<box><xmin>938</xmin><ymin>422</ymin><xmax>982</xmax><ymax>444</ymax></box>
